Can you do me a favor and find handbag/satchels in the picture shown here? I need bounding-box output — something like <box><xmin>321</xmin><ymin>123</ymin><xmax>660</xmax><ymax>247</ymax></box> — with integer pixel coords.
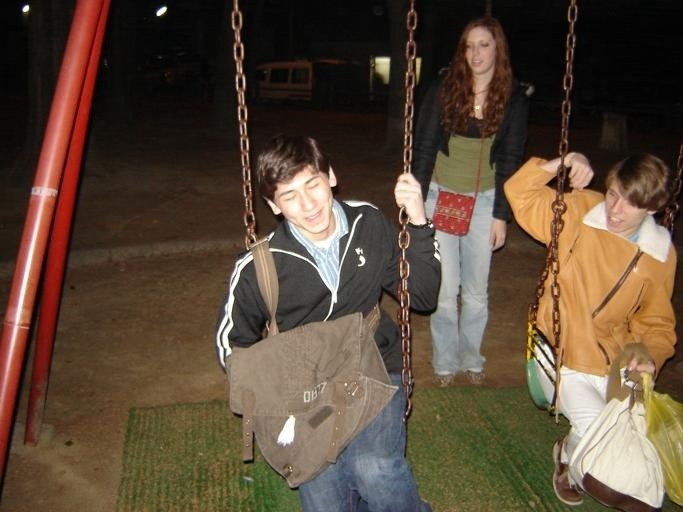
<box><xmin>429</xmin><ymin>180</ymin><xmax>475</xmax><ymax>240</ymax></box>
<box><xmin>222</xmin><ymin>237</ymin><xmax>400</xmax><ymax>490</ymax></box>
<box><xmin>568</xmin><ymin>343</ymin><xmax>666</xmax><ymax>511</ymax></box>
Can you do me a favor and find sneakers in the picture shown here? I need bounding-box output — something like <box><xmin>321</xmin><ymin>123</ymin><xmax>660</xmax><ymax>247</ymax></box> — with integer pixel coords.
<box><xmin>432</xmin><ymin>370</ymin><xmax>456</xmax><ymax>389</ymax></box>
<box><xmin>463</xmin><ymin>366</ymin><xmax>488</xmax><ymax>387</ymax></box>
<box><xmin>551</xmin><ymin>434</ymin><xmax>585</xmax><ymax>506</ymax></box>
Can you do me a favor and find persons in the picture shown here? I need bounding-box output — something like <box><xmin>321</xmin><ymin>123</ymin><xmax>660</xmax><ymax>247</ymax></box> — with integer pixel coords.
<box><xmin>212</xmin><ymin>131</ymin><xmax>441</xmax><ymax>509</ymax></box>
<box><xmin>398</xmin><ymin>15</ymin><xmax>531</xmax><ymax>388</ymax></box>
<box><xmin>504</xmin><ymin>152</ymin><xmax>678</xmax><ymax>511</ymax></box>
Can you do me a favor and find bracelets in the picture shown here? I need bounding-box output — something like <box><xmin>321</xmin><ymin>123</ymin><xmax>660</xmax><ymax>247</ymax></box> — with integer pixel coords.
<box><xmin>410</xmin><ymin>219</ymin><xmax>432</xmax><ymax>228</ymax></box>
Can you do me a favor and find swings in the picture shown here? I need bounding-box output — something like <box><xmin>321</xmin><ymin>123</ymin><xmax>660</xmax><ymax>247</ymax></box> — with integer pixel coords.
<box><xmin>526</xmin><ymin>1</ymin><xmax>683</xmax><ymax>422</ymax></box>
<box><xmin>230</xmin><ymin>0</ymin><xmax>414</xmax><ymax>420</ymax></box>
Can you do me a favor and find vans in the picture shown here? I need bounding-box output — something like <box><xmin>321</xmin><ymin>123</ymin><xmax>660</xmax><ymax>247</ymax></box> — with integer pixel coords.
<box><xmin>250</xmin><ymin>57</ymin><xmax>348</xmax><ymax>108</ymax></box>
